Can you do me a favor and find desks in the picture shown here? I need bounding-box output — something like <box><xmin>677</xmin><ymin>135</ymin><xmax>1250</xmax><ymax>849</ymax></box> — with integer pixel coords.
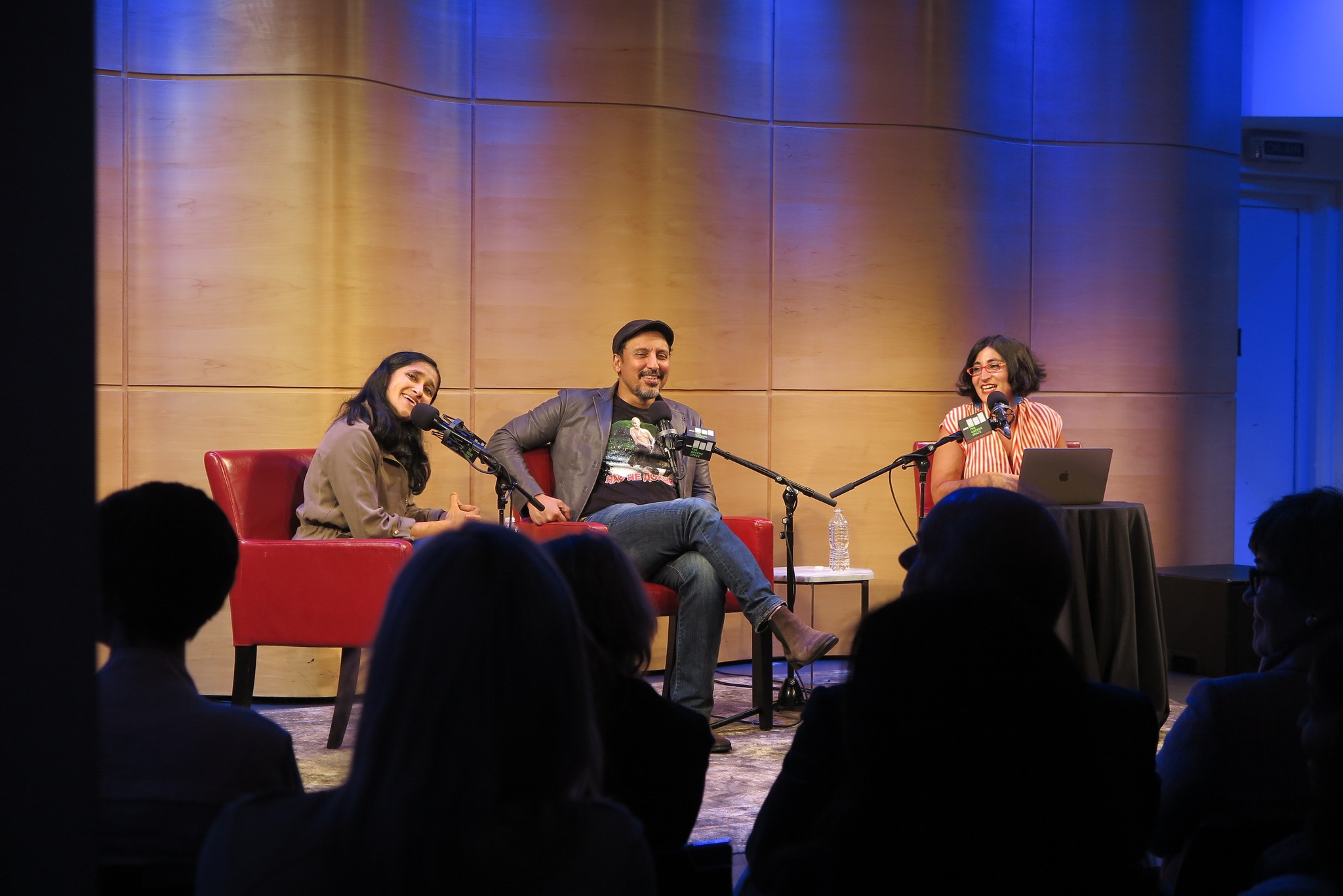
<box><xmin>772</xmin><ymin>564</ymin><xmax>875</xmax><ymax>694</ymax></box>
<box><xmin>1042</xmin><ymin>497</ymin><xmax>1174</xmax><ymax>730</ymax></box>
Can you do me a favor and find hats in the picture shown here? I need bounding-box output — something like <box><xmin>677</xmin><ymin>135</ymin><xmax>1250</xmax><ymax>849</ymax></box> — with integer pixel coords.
<box><xmin>612</xmin><ymin>320</ymin><xmax>674</xmax><ymax>353</ymax></box>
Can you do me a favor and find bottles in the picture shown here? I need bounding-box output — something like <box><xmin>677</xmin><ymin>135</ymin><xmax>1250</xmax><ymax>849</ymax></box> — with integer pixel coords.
<box><xmin>828</xmin><ymin>508</ymin><xmax>850</xmax><ymax>572</ymax></box>
<box><xmin>503</xmin><ymin>517</ymin><xmax>520</xmax><ymax>534</ymax></box>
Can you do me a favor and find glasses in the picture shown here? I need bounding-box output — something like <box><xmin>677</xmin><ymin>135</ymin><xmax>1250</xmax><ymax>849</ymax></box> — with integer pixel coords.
<box><xmin>967</xmin><ymin>362</ymin><xmax>1007</xmax><ymax>376</ymax></box>
<box><xmin>1249</xmin><ymin>567</ymin><xmax>1296</xmax><ymax>594</ymax></box>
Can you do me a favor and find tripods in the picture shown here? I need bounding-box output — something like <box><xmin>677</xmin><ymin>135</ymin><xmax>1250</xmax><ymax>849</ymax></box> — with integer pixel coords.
<box><xmin>672</xmin><ymin>425</ymin><xmax>836</xmax><ymax>729</ymax></box>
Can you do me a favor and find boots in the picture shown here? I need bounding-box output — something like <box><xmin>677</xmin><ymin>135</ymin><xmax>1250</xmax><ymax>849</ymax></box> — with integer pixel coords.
<box><xmin>709</xmin><ymin>729</ymin><xmax>732</xmax><ymax>753</ymax></box>
<box><xmin>770</xmin><ymin>607</ymin><xmax>839</xmax><ymax>670</ymax></box>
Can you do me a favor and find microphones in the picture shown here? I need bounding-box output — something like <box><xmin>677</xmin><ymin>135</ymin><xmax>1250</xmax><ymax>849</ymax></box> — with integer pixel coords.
<box><xmin>986</xmin><ymin>390</ymin><xmax>1011</xmax><ymax>440</ymax></box>
<box><xmin>649</xmin><ymin>400</ymin><xmax>680</xmax><ymax>480</ymax></box>
<box><xmin>409</xmin><ymin>402</ymin><xmax>495</xmax><ymax>459</ymax></box>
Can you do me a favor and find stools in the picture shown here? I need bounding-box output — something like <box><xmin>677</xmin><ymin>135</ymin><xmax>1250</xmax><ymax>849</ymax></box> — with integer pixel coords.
<box><xmin>1155</xmin><ymin>560</ymin><xmax>1262</xmax><ymax>678</ymax></box>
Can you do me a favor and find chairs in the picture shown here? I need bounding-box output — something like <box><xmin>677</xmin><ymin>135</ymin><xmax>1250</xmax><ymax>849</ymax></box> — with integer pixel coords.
<box><xmin>913</xmin><ymin>437</ymin><xmax>1084</xmax><ymax>535</ymax></box>
<box><xmin>499</xmin><ymin>442</ymin><xmax>779</xmax><ymax>735</ymax></box>
<box><xmin>202</xmin><ymin>444</ymin><xmax>414</xmax><ymax>752</ymax></box>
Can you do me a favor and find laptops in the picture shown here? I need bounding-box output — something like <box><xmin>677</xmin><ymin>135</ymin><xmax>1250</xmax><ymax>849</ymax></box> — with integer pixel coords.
<box><xmin>1017</xmin><ymin>448</ymin><xmax>1114</xmax><ymax>506</ymax></box>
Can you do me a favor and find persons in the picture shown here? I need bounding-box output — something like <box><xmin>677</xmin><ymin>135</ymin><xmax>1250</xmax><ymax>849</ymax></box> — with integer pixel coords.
<box><xmin>930</xmin><ymin>334</ymin><xmax>1068</xmax><ymax>506</ymax></box>
<box><xmin>734</xmin><ymin>487</ymin><xmax>1158</xmax><ymax>895</ymax></box>
<box><xmin>1150</xmin><ymin>486</ymin><xmax>1343</xmax><ymax>861</ymax></box>
<box><xmin>91</xmin><ymin>482</ymin><xmax>299</xmax><ymax>806</ymax></box>
<box><xmin>486</xmin><ymin>319</ymin><xmax>840</xmax><ymax>753</ymax></box>
<box><xmin>290</xmin><ymin>351</ymin><xmax>480</xmax><ymax>542</ymax></box>
<box><xmin>542</xmin><ymin>532</ymin><xmax>715</xmax><ymax>848</ymax></box>
<box><xmin>198</xmin><ymin>520</ymin><xmax>660</xmax><ymax>896</ymax></box>
